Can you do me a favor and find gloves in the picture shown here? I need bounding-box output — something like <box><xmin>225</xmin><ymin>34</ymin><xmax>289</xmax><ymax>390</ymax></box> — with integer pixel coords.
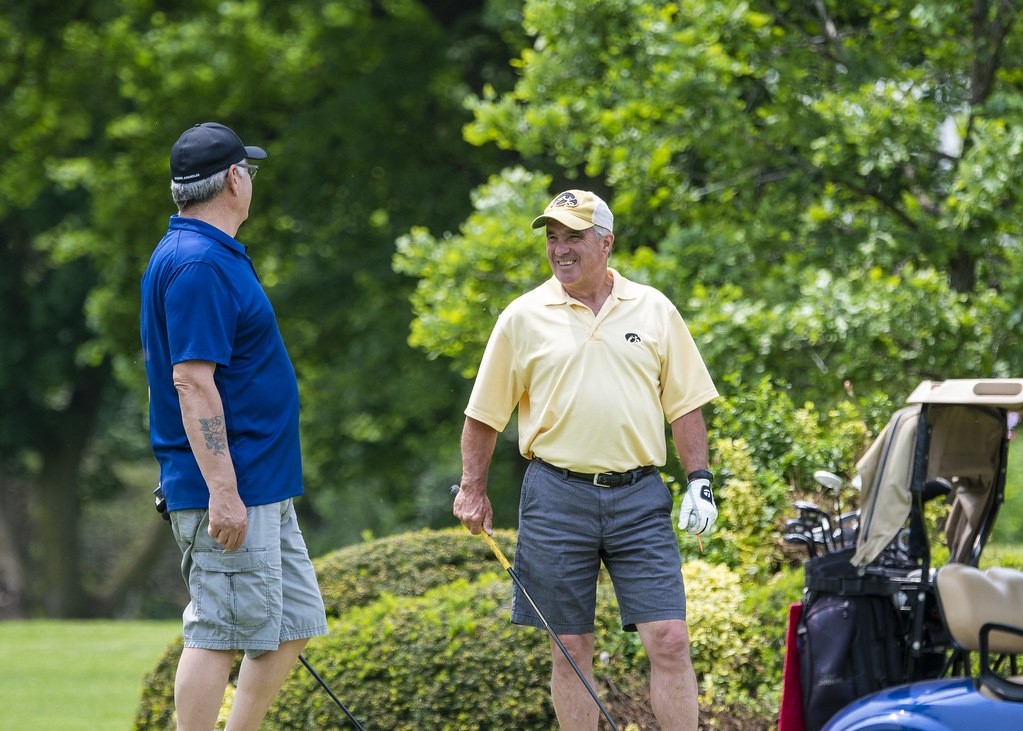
<box><xmin>678</xmin><ymin>470</ymin><xmax>718</xmax><ymax>535</ymax></box>
<box><xmin>155</xmin><ymin>484</ymin><xmax>171</xmax><ymax>525</ymax></box>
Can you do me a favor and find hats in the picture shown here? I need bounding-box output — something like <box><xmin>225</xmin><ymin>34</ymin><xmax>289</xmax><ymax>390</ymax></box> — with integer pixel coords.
<box><xmin>530</xmin><ymin>190</ymin><xmax>613</xmax><ymax>233</ymax></box>
<box><xmin>170</xmin><ymin>122</ymin><xmax>267</xmax><ymax>184</ymax></box>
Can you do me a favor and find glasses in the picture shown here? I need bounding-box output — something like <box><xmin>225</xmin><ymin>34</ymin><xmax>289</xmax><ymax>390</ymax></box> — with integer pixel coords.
<box><xmin>226</xmin><ymin>164</ymin><xmax>258</xmax><ymax>180</ymax></box>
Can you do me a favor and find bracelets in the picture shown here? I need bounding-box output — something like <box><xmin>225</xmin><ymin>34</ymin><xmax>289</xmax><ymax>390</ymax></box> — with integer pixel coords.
<box><xmin>687</xmin><ymin>469</ymin><xmax>713</xmax><ymax>483</ymax></box>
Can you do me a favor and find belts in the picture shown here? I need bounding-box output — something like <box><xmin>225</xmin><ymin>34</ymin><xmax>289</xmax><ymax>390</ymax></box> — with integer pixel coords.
<box><xmin>534</xmin><ymin>456</ymin><xmax>656</xmax><ymax>488</ymax></box>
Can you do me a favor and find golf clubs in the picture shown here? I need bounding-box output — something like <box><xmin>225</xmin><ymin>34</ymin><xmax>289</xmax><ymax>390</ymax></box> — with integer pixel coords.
<box><xmin>779</xmin><ymin>469</ymin><xmax>954</xmax><ymax>560</ymax></box>
<box><xmin>447</xmin><ymin>483</ymin><xmax>619</xmax><ymax>731</ymax></box>
<box><xmin>152</xmin><ymin>486</ymin><xmax>365</xmax><ymax>731</ymax></box>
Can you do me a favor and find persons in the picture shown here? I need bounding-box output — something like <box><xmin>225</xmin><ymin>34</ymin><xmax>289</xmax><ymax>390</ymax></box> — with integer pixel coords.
<box><xmin>138</xmin><ymin>121</ymin><xmax>327</xmax><ymax>731</ymax></box>
<box><xmin>452</xmin><ymin>189</ymin><xmax>721</xmax><ymax>731</ymax></box>
